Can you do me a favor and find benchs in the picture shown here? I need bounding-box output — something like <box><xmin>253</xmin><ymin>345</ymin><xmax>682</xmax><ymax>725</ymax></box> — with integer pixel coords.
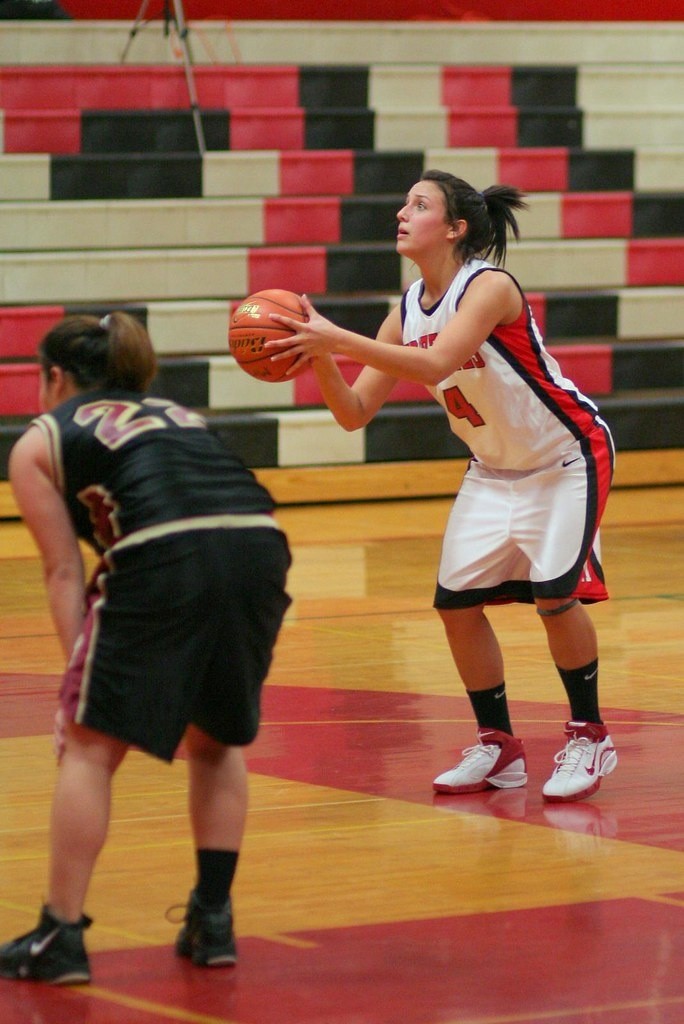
<box><xmin>0</xmin><ymin>22</ymin><xmax>684</xmax><ymax>460</ymax></box>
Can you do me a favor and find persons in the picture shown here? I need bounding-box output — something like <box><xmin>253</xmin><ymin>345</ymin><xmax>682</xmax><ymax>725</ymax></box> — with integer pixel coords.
<box><xmin>0</xmin><ymin>310</ymin><xmax>292</xmax><ymax>987</ymax></box>
<box><xmin>262</xmin><ymin>169</ymin><xmax>617</xmax><ymax>801</ymax></box>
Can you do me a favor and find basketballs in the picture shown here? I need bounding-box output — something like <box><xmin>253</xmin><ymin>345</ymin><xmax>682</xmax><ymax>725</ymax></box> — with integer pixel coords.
<box><xmin>229</xmin><ymin>289</ymin><xmax>312</xmax><ymax>383</ymax></box>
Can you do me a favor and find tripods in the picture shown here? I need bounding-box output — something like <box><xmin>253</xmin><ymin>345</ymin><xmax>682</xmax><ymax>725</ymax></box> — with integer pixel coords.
<box><xmin>119</xmin><ymin>0</ymin><xmax>207</xmax><ymax>159</ymax></box>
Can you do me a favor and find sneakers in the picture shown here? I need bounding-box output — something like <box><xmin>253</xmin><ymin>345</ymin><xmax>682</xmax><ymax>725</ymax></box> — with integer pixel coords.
<box><xmin>1</xmin><ymin>905</ymin><xmax>93</xmax><ymax>984</ymax></box>
<box><xmin>433</xmin><ymin>727</ymin><xmax>527</xmax><ymax>794</ymax></box>
<box><xmin>433</xmin><ymin>787</ymin><xmax>528</xmax><ymax>833</ymax></box>
<box><xmin>541</xmin><ymin>801</ymin><xmax>618</xmax><ymax>869</ymax></box>
<box><xmin>543</xmin><ymin>721</ymin><xmax>618</xmax><ymax>803</ymax></box>
<box><xmin>175</xmin><ymin>907</ymin><xmax>239</xmax><ymax>966</ymax></box>
